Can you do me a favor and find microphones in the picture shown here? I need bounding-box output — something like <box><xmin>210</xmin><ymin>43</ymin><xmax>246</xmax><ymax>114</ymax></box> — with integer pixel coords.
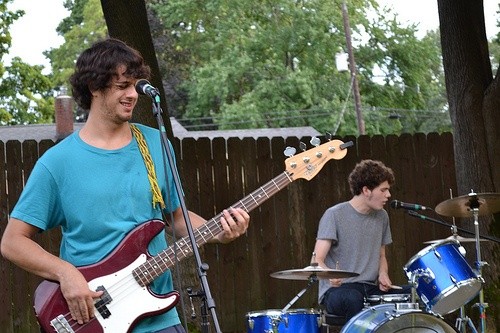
<box><xmin>388</xmin><ymin>200</ymin><xmax>431</xmax><ymax>212</ymax></box>
<box><xmin>134</xmin><ymin>79</ymin><xmax>161</xmax><ymax>99</ymax></box>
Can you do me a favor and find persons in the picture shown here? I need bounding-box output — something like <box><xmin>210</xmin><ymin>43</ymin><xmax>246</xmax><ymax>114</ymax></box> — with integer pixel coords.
<box><xmin>313</xmin><ymin>160</ymin><xmax>411</xmax><ymax>327</ymax></box>
<box><xmin>0</xmin><ymin>39</ymin><xmax>250</xmax><ymax>333</ymax></box>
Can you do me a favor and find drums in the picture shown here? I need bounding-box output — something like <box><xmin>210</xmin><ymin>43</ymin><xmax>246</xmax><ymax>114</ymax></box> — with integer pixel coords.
<box><xmin>339</xmin><ymin>303</ymin><xmax>461</xmax><ymax>333</ymax></box>
<box><xmin>403</xmin><ymin>240</ymin><xmax>484</xmax><ymax>316</ymax></box>
<box><xmin>363</xmin><ymin>293</ymin><xmax>422</xmax><ymax>310</ymax></box>
<box><xmin>246</xmin><ymin>307</ymin><xmax>322</xmax><ymax>333</ymax></box>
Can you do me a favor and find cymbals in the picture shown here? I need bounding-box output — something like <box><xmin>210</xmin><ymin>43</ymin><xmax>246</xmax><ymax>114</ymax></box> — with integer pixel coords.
<box><xmin>270</xmin><ymin>263</ymin><xmax>360</xmax><ymax>280</ymax></box>
<box><xmin>422</xmin><ymin>225</ymin><xmax>490</xmax><ymax>244</ymax></box>
<box><xmin>435</xmin><ymin>188</ymin><xmax>500</xmax><ymax>218</ymax></box>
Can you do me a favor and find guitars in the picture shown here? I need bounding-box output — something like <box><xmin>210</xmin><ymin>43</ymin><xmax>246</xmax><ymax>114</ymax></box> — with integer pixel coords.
<box><xmin>32</xmin><ymin>132</ymin><xmax>353</xmax><ymax>333</ymax></box>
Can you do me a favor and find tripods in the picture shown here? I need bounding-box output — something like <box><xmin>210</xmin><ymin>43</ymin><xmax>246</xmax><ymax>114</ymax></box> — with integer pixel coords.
<box><xmin>453</xmin><ymin>307</ymin><xmax>479</xmax><ymax>333</ymax></box>
